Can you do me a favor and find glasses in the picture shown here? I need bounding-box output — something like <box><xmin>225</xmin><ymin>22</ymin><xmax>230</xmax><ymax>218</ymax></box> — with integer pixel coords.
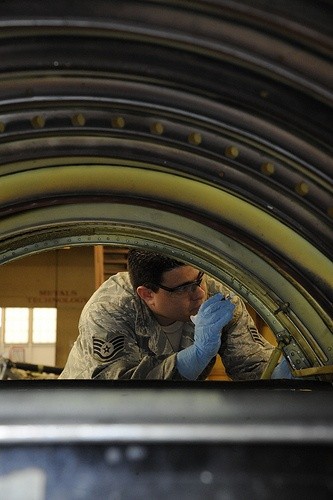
<box><xmin>149</xmin><ymin>271</ymin><xmax>205</xmax><ymax>295</ymax></box>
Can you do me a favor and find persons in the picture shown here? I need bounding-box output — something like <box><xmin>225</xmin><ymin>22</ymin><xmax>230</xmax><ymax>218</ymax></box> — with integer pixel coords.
<box><xmin>56</xmin><ymin>246</ymin><xmax>301</xmax><ymax>380</ymax></box>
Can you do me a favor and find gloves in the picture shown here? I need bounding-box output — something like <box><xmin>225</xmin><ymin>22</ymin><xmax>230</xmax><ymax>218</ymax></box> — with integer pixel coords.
<box><xmin>176</xmin><ymin>292</ymin><xmax>236</xmax><ymax>382</ymax></box>
<box><xmin>271</xmin><ymin>356</ymin><xmax>291</xmax><ymax>380</ymax></box>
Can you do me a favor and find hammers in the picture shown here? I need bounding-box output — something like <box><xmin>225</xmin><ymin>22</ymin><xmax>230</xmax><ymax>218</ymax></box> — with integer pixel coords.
<box><xmin>2</xmin><ymin>358</ymin><xmax>64</xmax><ymax>375</ymax></box>
